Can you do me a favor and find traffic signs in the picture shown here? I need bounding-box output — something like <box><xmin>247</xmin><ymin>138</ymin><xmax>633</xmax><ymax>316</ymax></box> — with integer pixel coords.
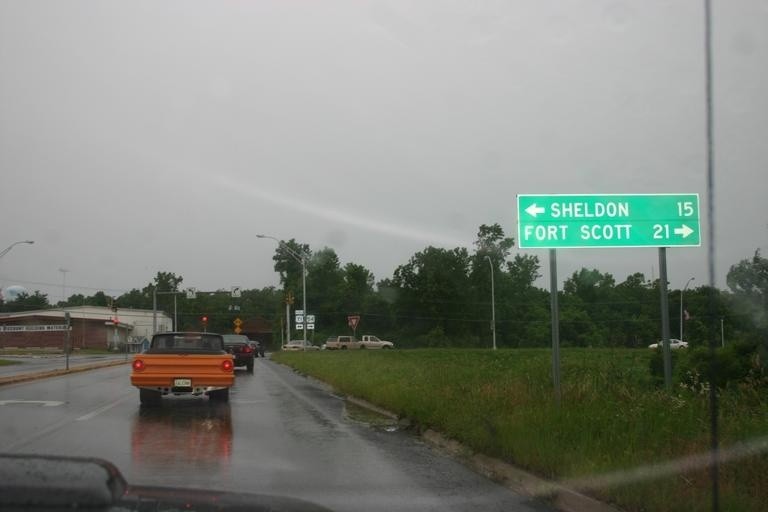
<box><xmin>516</xmin><ymin>194</ymin><xmax>702</xmax><ymax>248</ymax></box>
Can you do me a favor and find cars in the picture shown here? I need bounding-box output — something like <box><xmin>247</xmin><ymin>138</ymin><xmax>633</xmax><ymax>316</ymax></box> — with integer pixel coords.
<box><xmin>281</xmin><ymin>340</ymin><xmax>320</xmax><ymax>351</ymax></box>
<box><xmin>222</xmin><ymin>334</ymin><xmax>254</xmax><ymax>371</ymax></box>
<box><xmin>649</xmin><ymin>339</ymin><xmax>690</xmax><ymax>349</ymax></box>
<box><xmin>250</xmin><ymin>340</ymin><xmax>265</xmax><ymax>357</ymax></box>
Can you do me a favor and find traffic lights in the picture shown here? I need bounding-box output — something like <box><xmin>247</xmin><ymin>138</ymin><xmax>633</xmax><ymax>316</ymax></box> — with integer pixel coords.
<box><xmin>202</xmin><ymin>316</ymin><xmax>208</xmax><ymax>322</ymax></box>
<box><xmin>114</xmin><ymin>319</ymin><xmax>118</xmax><ymax>324</ymax></box>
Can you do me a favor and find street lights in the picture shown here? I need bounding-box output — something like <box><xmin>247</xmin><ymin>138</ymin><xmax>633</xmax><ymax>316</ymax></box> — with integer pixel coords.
<box><xmin>484</xmin><ymin>255</ymin><xmax>496</xmax><ymax>348</ymax></box>
<box><xmin>680</xmin><ymin>276</ymin><xmax>696</xmax><ymax>342</ymax></box>
<box><xmin>1</xmin><ymin>240</ymin><xmax>35</xmax><ymax>259</ymax></box>
<box><xmin>255</xmin><ymin>234</ymin><xmax>308</xmax><ymax>351</ymax></box>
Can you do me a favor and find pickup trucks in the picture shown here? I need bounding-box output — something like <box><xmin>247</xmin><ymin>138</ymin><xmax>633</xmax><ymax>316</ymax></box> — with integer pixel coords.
<box><xmin>326</xmin><ymin>335</ymin><xmax>360</xmax><ymax>350</ymax></box>
<box><xmin>130</xmin><ymin>331</ymin><xmax>236</xmax><ymax>405</ymax></box>
<box><xmin>359</xmin><ymin>335</ymin><xmax>394</xmax><ymax>350</ymax></box>
